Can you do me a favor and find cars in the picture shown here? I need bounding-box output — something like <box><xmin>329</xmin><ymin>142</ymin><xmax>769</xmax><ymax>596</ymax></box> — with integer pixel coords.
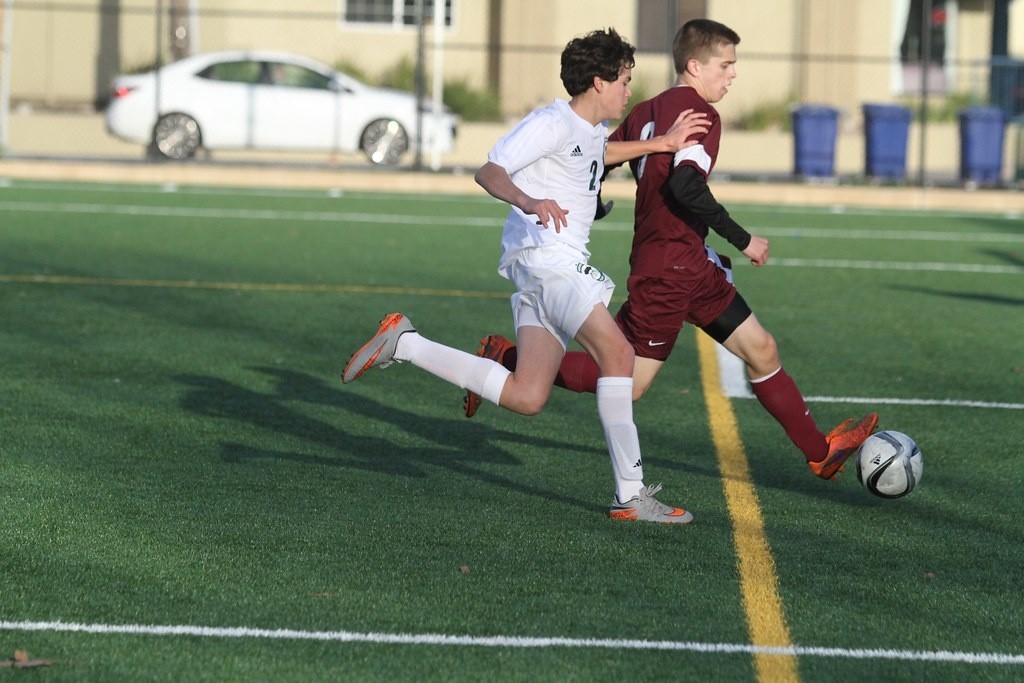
<box><xmin>105</xmin><ymin>49</ymin><xmax>458</xmax><ymax>170</ymax></box>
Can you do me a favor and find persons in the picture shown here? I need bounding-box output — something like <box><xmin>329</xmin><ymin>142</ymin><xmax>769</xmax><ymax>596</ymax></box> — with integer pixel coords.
<box><xmin>338</xmin><ymin>26</ymin><xmax>713</xmax><ymax>524</ymax></box>
<box><xmin>461</xmin><ymin>18</ymin><xmax>879</xmax><ymax>481</ymax></box>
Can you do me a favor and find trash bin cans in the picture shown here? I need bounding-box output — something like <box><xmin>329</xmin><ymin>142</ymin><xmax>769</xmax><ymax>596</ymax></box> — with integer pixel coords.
<box><xmin>793</xmin><ymin>105</ymin><xmax>840</xmax><ymax>177</ymax></box>
<box><xmin>863</xmin><ymin>103</ymin><xmax>910</xmax><ymax>177</ymax></box>
<box><xmin>990</xmin><ymin>56</ymin><xmax>1024</xmax><ymax>120</ymax></box>
<box><xmin>958</xmin><ymin>104</ymin><xmax>1004</xmax><ymax>183</ymax></box>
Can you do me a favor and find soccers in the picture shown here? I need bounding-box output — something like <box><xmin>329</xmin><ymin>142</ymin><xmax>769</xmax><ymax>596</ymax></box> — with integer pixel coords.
<box><xmin>856</xmin><ymin>430</ymin><xmax>924</xmax><ymax>500</ymax></box>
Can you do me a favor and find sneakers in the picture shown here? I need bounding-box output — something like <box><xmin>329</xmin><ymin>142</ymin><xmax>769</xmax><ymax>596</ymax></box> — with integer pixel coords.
<box><xmin>342</xmin><ymin>311</ymin><xmax>417</xmax><ymax>385</ymax></box>
<box><xmin>610</xmin><ymin>483</ymin><xmax>694</xmax><ymax>525</ymax></box>
<box><xmin>462</xmin><ymin>332</ymin><xmax>513</xmax><ymax>417</ymax></box>
<box><xmin>809</xmin><ymin>412</ymin><xmax>880</xmax><ymax>480</ymax></box>
